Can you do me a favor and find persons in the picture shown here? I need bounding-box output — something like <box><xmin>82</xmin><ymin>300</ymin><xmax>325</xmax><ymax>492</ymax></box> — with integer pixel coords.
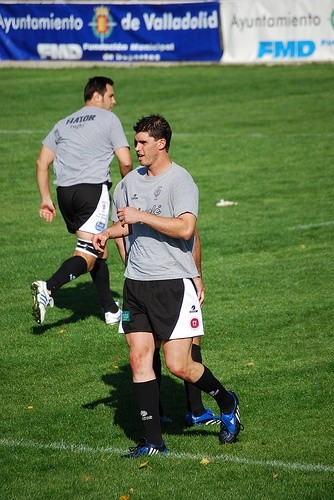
<box><xmin>92</xmin><ymin>114</ymin><xmax>242</xmax><ymax>458</ymax></box>
<box><xmin>30</xmin><ymin>76</ymin><xmax>134</xmax><ymax>325</ymax></box>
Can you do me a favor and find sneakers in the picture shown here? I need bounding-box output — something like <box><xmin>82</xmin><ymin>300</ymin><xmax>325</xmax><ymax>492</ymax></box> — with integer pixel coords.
<box><xmin>105</xmin><ymin>302</ymin><xmax>122</xmax><ymax>324</ymax></box>
<box><xmin>122</xmin><ymin>437</ymin><xmax>167</xmax><ymax>459</ymax></box>
<box><xmin>31</xmin><ymin>281</ymin><xmax>54</xmax><ymax>324</ymax></box>
<box><xmin>184</xmin><ymin>408</ymin><xmax>221</xmax><ymax>427</ymax></box>
<box><xmin>219</xmin><ymin>391</ymin><xmax>244</xmax><ymax>444</ymax></box>
<box><xmin>159</xmin><ymin>415</ymin><xmax>173</xmax><ymax>424</ymax></box>
